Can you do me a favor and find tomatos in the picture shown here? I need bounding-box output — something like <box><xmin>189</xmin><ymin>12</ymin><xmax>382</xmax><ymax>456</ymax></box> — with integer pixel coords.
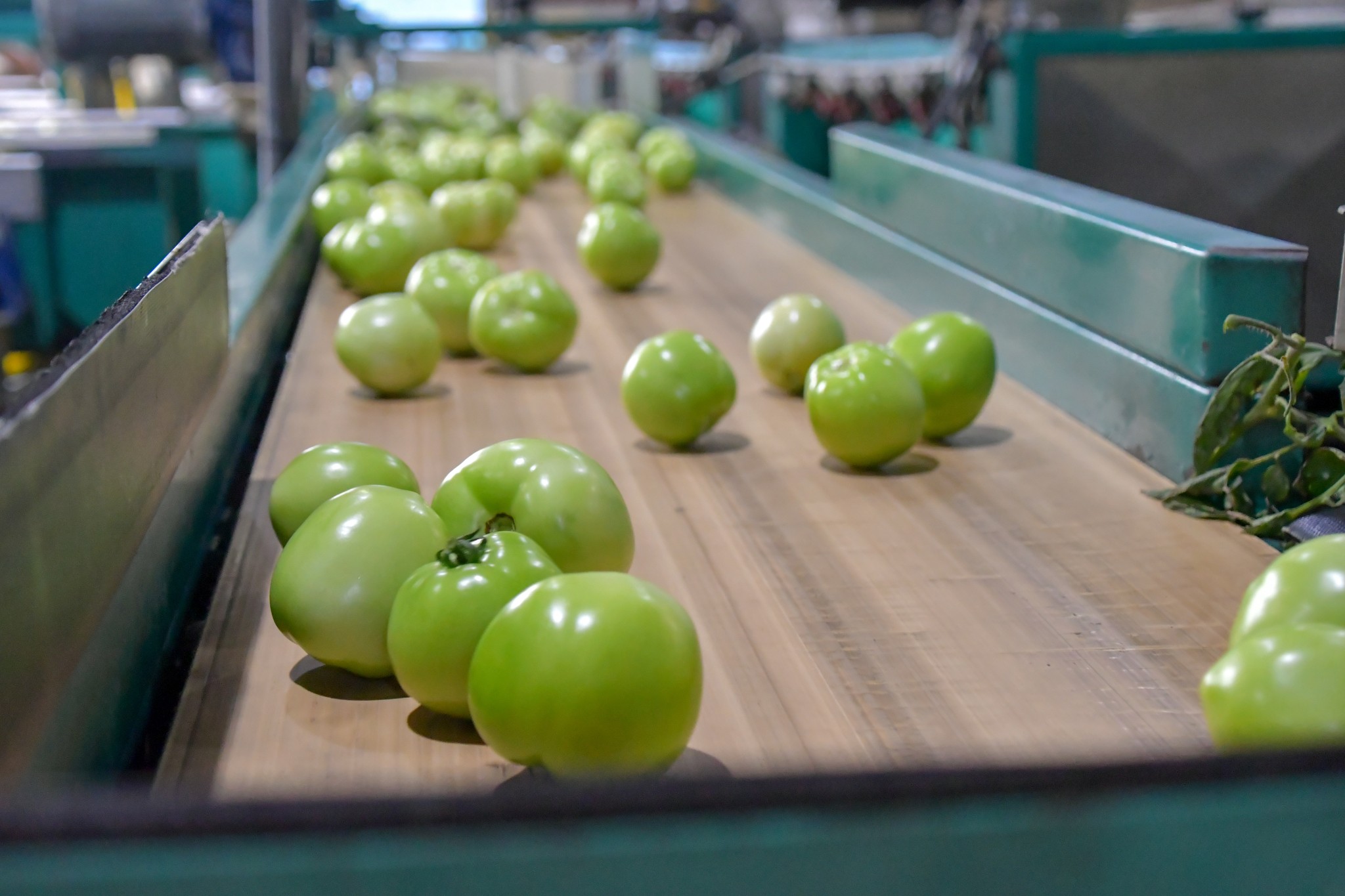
<box><xmin>796</xmin><ymin>339</ymin><xmax>926</xmax><ymax>473</ymax></box>
<box><xmin>265</xmin><ymin>482</ymin><xmax>448</xmax><ymax>681</ymax></box>
<box><xmin>292</xmin><ymin>60</ymin><xmax>703</xmax><ymax>291</ymax></box>
<box><xmin>429</xmin><ymin>436</ymin><xmax>632</xmax><ymax>574</ymax></box>
<box><xmin>466</xmin><ymin>267</ymin><xmax>582</xmax><ymax>377</ymax></box>
<box><xmin>332</xmin><ymin>293</ymin><xmax>439</xmax><ymax>400</ymax></box>
<box><xmin>622</xmin><ymin>328</ymin><xmax>737</xmax><ymax>450</ymax></box>
<box><xmin>269</xmin><ymin>438</ymin><xmax>420</xmax><ymax>551</ymax></box>
<box><xmin>1228</xmin><ymin>534</ymin><xmax>1345</xmax><ymax>645</ymax></box>
<box><xmin>462</xmin><ymin>576</ymin><xmax>706</xmax><ymax>782</ymax></box>
<box><xmin>397</xmin><ymin>247</ymin><xmax>498</xmax><ymax>358</ymax></box>
<box><xmin>886</xmin><ymin>309</ymin><xmax>995</xmax><ymax>446</ymax></box>
<box><xmin>1188</xmin><ymin>622</ymin><xmax>1345</xmax><ymax>755</ymax></box>
<box><xmin>385</xmin><ymin>528</ymin><xmax>567</xmax><ymax>719</ymax></box>
<box><xmin>743</xmin><ymin>289</ymin><xmax>848</xmax><ymax>400</ymax></box>
